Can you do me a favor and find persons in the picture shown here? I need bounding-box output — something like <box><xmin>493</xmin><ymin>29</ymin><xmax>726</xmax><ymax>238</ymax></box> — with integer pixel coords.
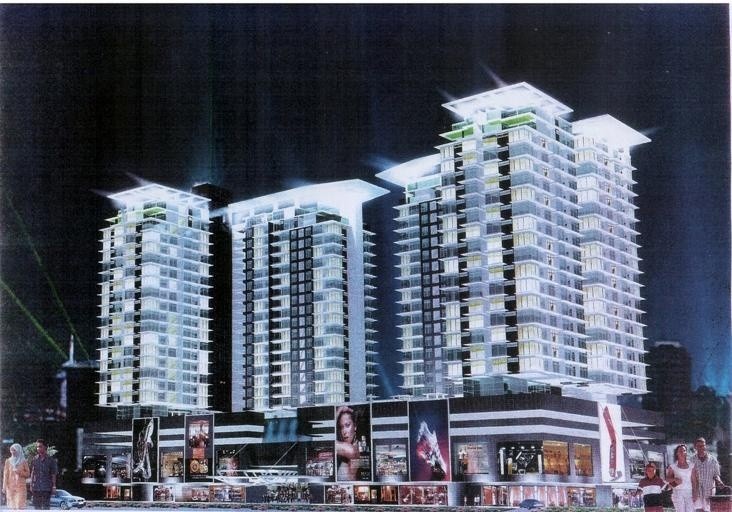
<box><xmin>263</xmin><ymin>488</ymin><xmax>311</xmax><ymax>504</ymax></box>
<box><xmin>473</xmin><ymin>494</ymin><xmax>476</xmax><ymax>506</ymax></box>
<box><xmin>463</xmin><ymin>495</ymin><xmax>468</xmax><ymax>506</ymax></box>
<box><xmin>189</xmin><ymin>435</ymin><xmax>210</xmax><ymax>448</ymax></box>
<box><xmin>690</xmin><ymin>437</ymin><xmax>725</xmax><ymax>512</ymax></box>
<box><xmin>668</xmin><ymin>445</ymin><xmax>697</xmax><ymax>512</ymax></box>
<box><xmin>632</xmin><ymin>463</ymin><xmax>680</xmax><ymax>512</ymax></box>
<box><xmin>98</xmin><ymin>465</ymin><xmax>107</xmax><ymax>482</ymax></box>
<box><xmin>30</xmin><ymin>439</ymin><xmax>57</xmax><ymax>510</ymax></box>
<box><xmin>458</xmin><ymin>448</ymin><xmax>464</xmax><ymax>474</ymax></box>
<box><xmin>477</xmin><ymin>493</ymin><xmax>481</xmax><ymax>506</ymax></box>
<box><xmin>2</xmin><ymin>444</ymin><xmax>29</xmax><ymax>510</ymax></box>
<box><xmin>336</xmin><ymin>407</ymin><xmax>361</xmax><ymax>481</ymax></box>
